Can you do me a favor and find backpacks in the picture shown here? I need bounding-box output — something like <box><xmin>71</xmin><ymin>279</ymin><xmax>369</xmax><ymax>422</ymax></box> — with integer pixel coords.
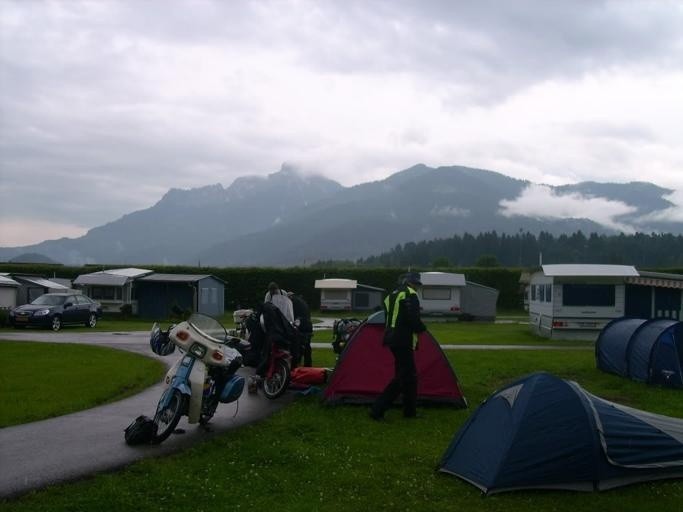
<box><xmin>124</xmin><ymin>414</ymin><xmax>158</xmax><ymax>446</ymax></box>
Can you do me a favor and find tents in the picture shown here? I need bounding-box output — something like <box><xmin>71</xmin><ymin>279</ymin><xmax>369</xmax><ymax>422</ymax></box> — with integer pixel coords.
<box><xmin>594</xmin><ymin>314</ymin><xmax>683</xmax><ymax>391</ymax></box>
<box><xmin>435</xmin><ymin>374</ymin><xmax>683</xmax><ymax>499</ymax></box>
<box><xmin>320</xmin><ymin>310</ymin><xmax>467</xmax><ymax>409</ymax></box>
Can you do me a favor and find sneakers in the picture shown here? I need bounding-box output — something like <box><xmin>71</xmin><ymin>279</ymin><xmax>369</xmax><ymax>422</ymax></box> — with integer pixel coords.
<box><xmin>369</xmin><ymin>409</ymin><xmax>385</xmax><ymax>422</ymax></box>
<box><xmin>403</xmin><ymin>411</ymin><xmax>422</xmax><ymax>419</ymax></box>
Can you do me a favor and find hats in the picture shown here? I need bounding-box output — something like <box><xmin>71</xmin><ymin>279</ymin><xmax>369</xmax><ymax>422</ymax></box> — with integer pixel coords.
<box><xmin>405</xmin><ymin>272</ymin><xmax>422</xmax><ymax>286</ymax></box>
<box><xmin>287</xmin><ymin>292</ymin><xmax>295</xmax><ymax>297</ymax></box>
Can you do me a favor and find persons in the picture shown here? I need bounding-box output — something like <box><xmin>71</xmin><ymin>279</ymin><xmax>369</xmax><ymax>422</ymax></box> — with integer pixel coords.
<box><xmin>264</xmin><ymin>282</ymin><xmax>287</xmax><ymax>304</ymax></box>
<box><xmin>287</xmin><ymin>292</ymin><xmax>312</xmax><ymax>368</ymax></box>
<box><xmin>368</xmin><ymin>271</ymin><xmax>425</xmax><ymax>421</ymax></box>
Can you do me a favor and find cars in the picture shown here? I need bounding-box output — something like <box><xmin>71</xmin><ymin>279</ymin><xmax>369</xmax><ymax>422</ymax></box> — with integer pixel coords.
<box><xmin>9</xmin><ymin>293</ymin><xmax>103</xmax><ymax>332</ymax></box>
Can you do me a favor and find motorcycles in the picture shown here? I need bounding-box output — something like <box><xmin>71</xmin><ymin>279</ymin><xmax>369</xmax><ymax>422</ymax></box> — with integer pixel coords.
<box><xmin>154</xmin><ymin>309</ymin><xmax>301</xmax><ymax>443</ymax></box>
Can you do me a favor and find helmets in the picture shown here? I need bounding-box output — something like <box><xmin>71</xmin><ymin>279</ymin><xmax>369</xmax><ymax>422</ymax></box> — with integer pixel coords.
<box><xmin>150</xmin><ymin>322</ymin><xmax>175</xmax><ymax>356</ymax></box>
